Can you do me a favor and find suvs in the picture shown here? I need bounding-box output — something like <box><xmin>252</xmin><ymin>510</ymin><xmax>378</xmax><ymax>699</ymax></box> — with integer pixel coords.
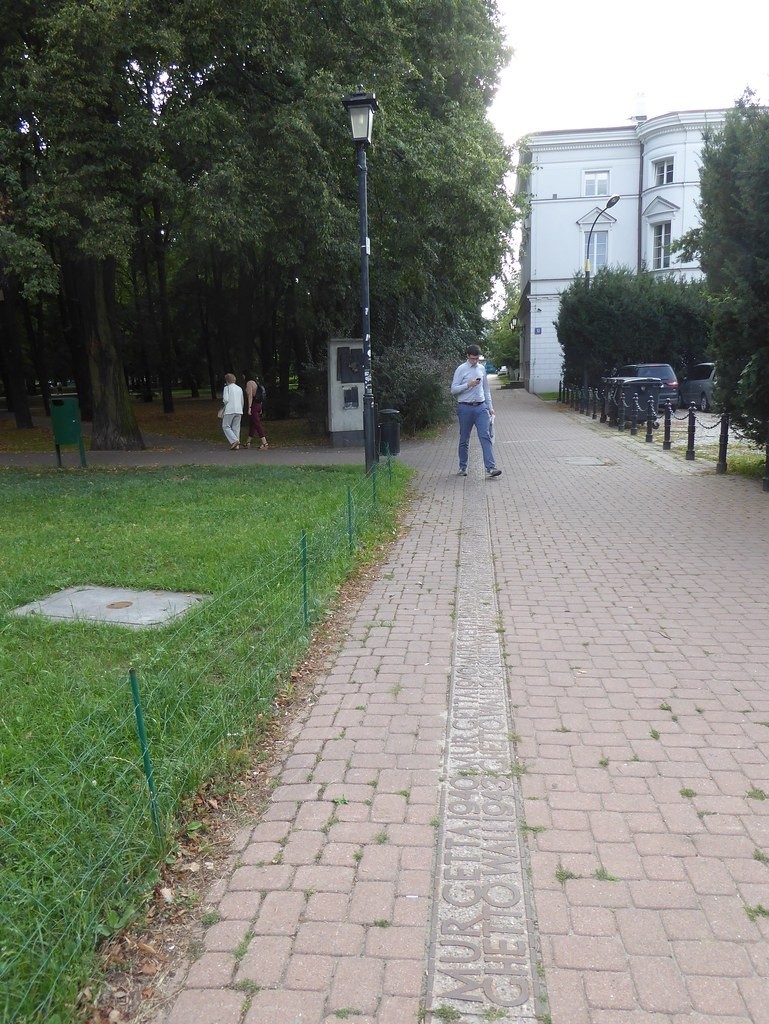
<box><xmin>612</xmin><ymin>363</ymin><xmax>679</xmax><ymax>413</ymax></box>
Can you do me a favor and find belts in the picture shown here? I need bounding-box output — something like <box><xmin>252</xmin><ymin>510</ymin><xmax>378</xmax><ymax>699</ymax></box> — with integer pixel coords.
<box><xmin>458</xmin><ymin>402</ymin><xmax>483</xmax><ymax>406</ymax></box>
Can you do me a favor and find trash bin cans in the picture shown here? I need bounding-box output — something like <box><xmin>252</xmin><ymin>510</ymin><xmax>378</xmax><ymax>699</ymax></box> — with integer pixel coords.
<box><xmin>378</xmin><ymin>409</ymin><xmax>400</xmax><ymax>457</ymax></box>
<box><xmin>604</xmin><ymin>377</ymin><xmax>662</xmax><ymax>423</ymax></box>
<box><xmin>48</xmin><ymin>398</ymin><xmax>81</xmax><ymax>445</ymax></box>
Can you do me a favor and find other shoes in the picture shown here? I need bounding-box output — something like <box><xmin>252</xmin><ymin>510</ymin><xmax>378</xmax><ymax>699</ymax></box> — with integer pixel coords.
<box><xmin>487</xmin><ymin>468</ymin><xmax>501</xmax><ymax>477</ymax></box>
<box><xmin>257</xmin><ymin>443</ymin><xmax>269</xmax><ymax>449</ymax></box>
<box><xmin>229</xmin><ymin>441</ymin><xmax>240</xmax><ymax>449</ymax></box>
<box><xmin>242</xmin><ymin>442</ymin><xmax>250</xmax><ymax>449</ymax></box>
<box><xmin>459</xmin><ymin>467</ymin><xmax>467</xmax><ymax>476</ymax></box>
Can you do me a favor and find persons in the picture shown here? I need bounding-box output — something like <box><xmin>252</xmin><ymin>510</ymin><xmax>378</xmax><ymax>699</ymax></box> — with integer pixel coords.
<box><xmin>241</xmin><ymin>369</ymin><xmax>269</xmax><ymax>450</ymax></box>
<box><xmin>451</xmin><ymin>346</ymin><xmax>502</xmax><ymax>477</ymax></box>
<box><xmin>222</xmin><ymin>373</ymin><xmax>244</xmax><ymax>450</ymax></box>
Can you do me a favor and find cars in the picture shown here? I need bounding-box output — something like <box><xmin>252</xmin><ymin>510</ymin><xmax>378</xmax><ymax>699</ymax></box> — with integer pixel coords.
<box><xmin>498</xmin><ymin>365</ymin><xmax>507</xmax><ymax>376</ymax></box>
<box><xmin>677</xmin><ymin>362</ymin><xmax>718</xmax><ymax>413</ymax></box>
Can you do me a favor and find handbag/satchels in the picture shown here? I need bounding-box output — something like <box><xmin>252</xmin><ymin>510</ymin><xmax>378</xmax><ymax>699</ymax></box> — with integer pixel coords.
<box><xmin>489</xmin><ymin>414</ymin><xmax>495</xmax><ymax>445</ymax></box>
<box><xmin>217</xmin><ymin>407</ymin><xmax>225</xmax><ymax>418</ymax></box>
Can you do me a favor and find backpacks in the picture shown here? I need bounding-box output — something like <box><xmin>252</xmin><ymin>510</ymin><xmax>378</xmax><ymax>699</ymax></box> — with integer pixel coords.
<box><xmin>251</xmin><ymin>380</ymin><xmax>266</xmax><ymax>403</ymax></box>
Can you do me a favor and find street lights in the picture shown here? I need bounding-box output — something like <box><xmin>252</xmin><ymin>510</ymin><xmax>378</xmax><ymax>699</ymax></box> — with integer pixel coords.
<box><xmin>583</xmin><ymin>193</ymin><xmax>621</xmax><ymax>288</ymax></box>
<box><xmin>342</xmin><ymin>80</ymin><xmax>380</xmax><ymax>473</ymax></box>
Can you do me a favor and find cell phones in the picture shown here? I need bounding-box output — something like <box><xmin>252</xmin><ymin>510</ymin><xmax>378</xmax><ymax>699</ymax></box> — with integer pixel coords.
<box><xmin>476</xmin><ymin>378</ymin><xmax>481</xmax><ymax>381</ymax></box>
